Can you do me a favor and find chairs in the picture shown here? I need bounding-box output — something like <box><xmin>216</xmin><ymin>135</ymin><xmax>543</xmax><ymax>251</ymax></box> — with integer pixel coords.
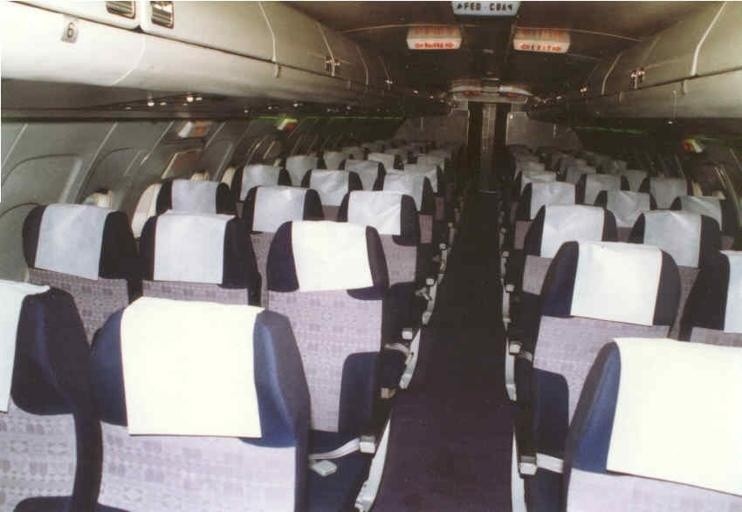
<box><xmin>510</xmin><ymin>337</ymin><xmax>740</xmax><ymax>511</ymax></box>
<box><xmin>155</xmin><ymin>137</ymin><xmax>471</xmax><ymax>400</ymax></box>
<box><xmin>91</xmin><ymin>295</ymin><xmax>311</xmax><ymax>512</ymax></box>
<box><xmin>497</xmin><ymin>143</ymin><xmax>739</xmax><ymax>402</ymax></box>
<box><xmin>1</xmin><ymin>278</ymin><xmax>90</xmax><ymax>512</ymax></box>
<box><xmin>509</xmin><ymin>241</ymin><xmax>682</xmax><ymax>512</ymax></box>
<box><xmin>265</xmin><ymin>220</ymin><xmax>399</xmax><ymax>469</ymax></box>
<box><xmin>139</xmin><ymin>211</ymin><xmax>259</xmax><ymax>306</ymax></box>
<box><xmin>22</xmin><ymin>204</ymin><xmax>136</xmax><ymax>346</ymax></box>
<box><xmin>678</xmin><ymin>249</ymin><xmax>741</xmax><ymax>345</ymax></box>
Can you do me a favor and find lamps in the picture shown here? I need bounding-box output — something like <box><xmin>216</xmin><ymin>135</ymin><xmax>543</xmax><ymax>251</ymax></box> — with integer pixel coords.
<box><xmin>405</xmin><ymin>24</ymin><xmax>462</xmax><ymax>38</ymax></box>
<box><xmin>407</xmin><ymin>38</ymin><xmax>462</xmax><ymax>52</ymax></box>
<box><xmin>514</xmin><ymin>26</ymin><xmax>571</xmax><ymax>42</ymax></box>
<box><xmin>509</xmin><ymin>42</ymin><xmax>573</xmax><ymax>54</ymax></box>
